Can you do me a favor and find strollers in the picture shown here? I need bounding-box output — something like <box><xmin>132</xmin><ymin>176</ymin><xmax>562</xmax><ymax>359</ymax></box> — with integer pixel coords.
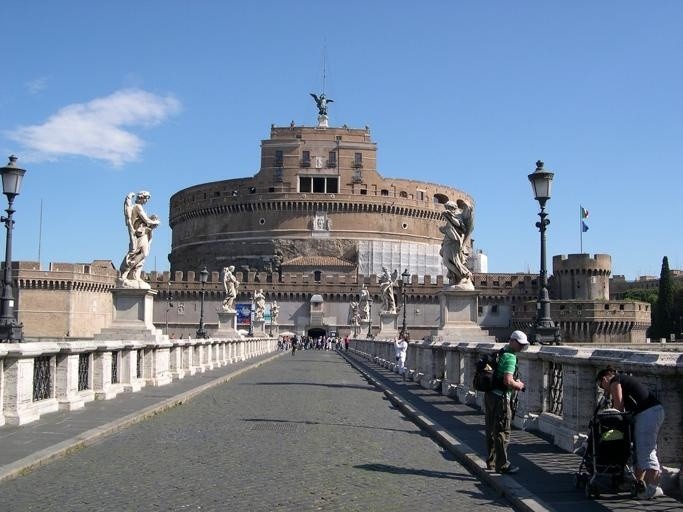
<box><xmin>573</xmin><ymin>395</ymin><xmax>647</xmax><ymax>500</ymax></box>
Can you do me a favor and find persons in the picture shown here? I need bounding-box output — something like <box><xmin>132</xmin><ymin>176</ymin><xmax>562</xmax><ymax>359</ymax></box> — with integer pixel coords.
<box><xmin>120</xmin><ymin>190</ymin><xmax>160</xmax><ymax>283</ymax></box>
<box><xmin>357</xmin><ymin>285</ymin><xmax>372</xmax><ymax>319</ymax></box>
<box><xmin>256</xmin><ymin>289</ymin><xmax>266</xmax><ymax>321</ymax></box>
<box><xmin>440</xmin><ymin>201</ymin><xmax>467</xmax><ymax>286</ymax></box>
<box><xmin>320</xmin><ymin>95</ymin><xmax>328</xmax><ymax>115</ymax></box>
<box><xmin>271</xmin><ymin>301</ymin><xmax>280</xmax><ymax>320</ymax></box>
<box><xmin>481</xmin><ymin>329</ymin><xmax>530</xmax><ymax>475</ymax></box>
<box><xmin>595</xmin><ymin>366</ymin><xmax>666</xmax><ymax>500</ymax></box>
<box><xmin>349</xmin><ymin>301</ymin><xmax>358</xmax><ymax>325</ymax></box>
<box><xmin>222</xmin><ymin>264</ymin><xmax>240</xmax><ymax>312</ymax></box>
<box><xmin>279</xmin><ymin>335</ymin><xmax>349</xmax><ymax>351</ymax></box>
<box><xmin>393</xmin><ymin>333</ymin><xmax>409</xmax><ymax>378</ymax></box>
<box><xmin>290</xmin><ymin>335</ymin><xmax>298</xmax><ymax>356</ymax></box>
<box><xmin>377</xmin><ymin>266</ymin><xmax>399</xmax><ymax>314</ymax></box>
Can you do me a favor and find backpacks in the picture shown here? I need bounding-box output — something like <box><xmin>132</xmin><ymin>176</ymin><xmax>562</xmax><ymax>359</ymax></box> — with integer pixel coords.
<box><xmin>471</xmin><ymin>348</ymin><xmax>513</xmax><ymax>392</ymax></box>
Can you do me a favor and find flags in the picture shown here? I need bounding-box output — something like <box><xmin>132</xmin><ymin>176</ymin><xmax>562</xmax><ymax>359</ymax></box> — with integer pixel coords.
<box><xmin>580</xmin><ymin>207</ymin><xmax>589</xmax><ymax>218</ymax></box>
<box><xmin>581</xmin><ymin>219</ymin><xmax>589</xmax><ymax>233</ymax></box>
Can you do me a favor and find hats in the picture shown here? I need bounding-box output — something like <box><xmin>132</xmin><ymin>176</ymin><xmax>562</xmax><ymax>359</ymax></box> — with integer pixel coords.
<box><xmin>509</xmin><ymin>330</ymin><xmax>530</xmax><ymax>347</ymax></box>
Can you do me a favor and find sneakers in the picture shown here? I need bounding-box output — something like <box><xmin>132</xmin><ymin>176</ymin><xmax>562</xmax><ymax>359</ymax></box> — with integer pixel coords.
<box><xmin>486</xmin><ymin>462</ymin><xmax>520</xmax><ymax>475</ymax></box>
<box><xmin>636</xmin><ymin>484</ymin><xmax>664</xmax><ymax>501</ymax></box>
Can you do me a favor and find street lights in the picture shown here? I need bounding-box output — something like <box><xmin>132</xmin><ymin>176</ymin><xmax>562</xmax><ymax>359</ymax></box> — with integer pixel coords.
<box><xmin>0</xmin><ymin>155</ymin><xmax>27</xmax><ymax>343</ymax></box>
<box><xmin>367</xmin><ymin>299</ymin><xmax>373</xmax><ymax>338</ymax></box>
<box><xmin>528</xmin><ymin>160</ymin><xmax>562</xmax><ymax>345</ymax></box>
<box><xmin>269</xmin><ymin>307</ymin><xmax>274</xmax><ymax>337</ymax></box>
<box><xmin>399</xmin><ymin>268</ymin><xmax>410</xmax><ymax>340</ymax></box>
<box><xmin>196</xmin><ymin>266</ymin><xmax>209</xmax><ymax>339</ymax></box>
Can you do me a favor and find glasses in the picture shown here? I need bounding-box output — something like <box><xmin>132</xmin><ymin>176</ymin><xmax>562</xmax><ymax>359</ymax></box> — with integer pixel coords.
<box><xmin>599</xmin><ymin>379</ymin><xmax>605</xmax><ymax>389</ymax></box>
